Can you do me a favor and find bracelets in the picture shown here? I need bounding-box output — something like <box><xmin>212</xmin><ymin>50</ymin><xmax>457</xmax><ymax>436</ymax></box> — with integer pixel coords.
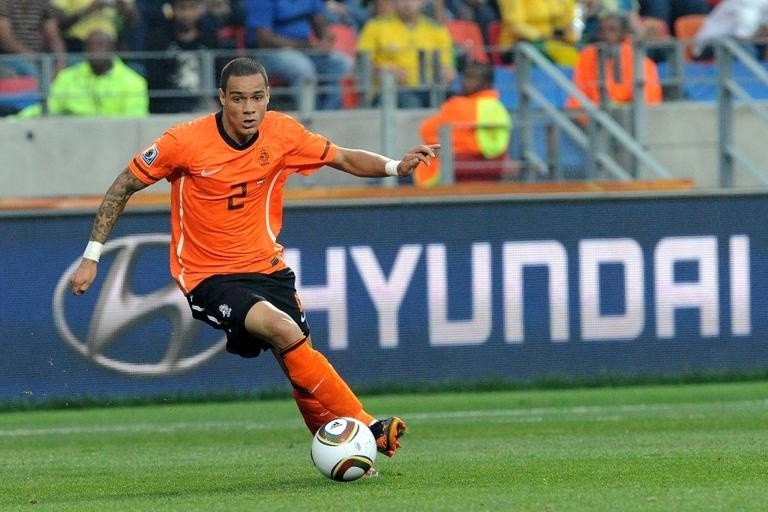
<box><xmin>82</xmin><ymin>239</ymin><xmax>104</xmax><ymax>263</ymax></box>
<box><xmin>384</xmin><ymin>159</ymin><xmax>401</xmax><ymax>177</ymax></box>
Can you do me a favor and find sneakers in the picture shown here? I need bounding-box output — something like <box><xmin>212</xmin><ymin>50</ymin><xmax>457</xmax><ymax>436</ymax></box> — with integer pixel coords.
<box><xmin>368</xmin><ymin>416</ymin><xmax>407</xmax><ymax>458</ymax></box>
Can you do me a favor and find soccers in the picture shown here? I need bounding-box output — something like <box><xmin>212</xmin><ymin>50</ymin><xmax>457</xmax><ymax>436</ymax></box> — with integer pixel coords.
<box><xmin>311</xmin><ymin>416</ymin><xmax>377</xmax><ymax>482</ymax></box>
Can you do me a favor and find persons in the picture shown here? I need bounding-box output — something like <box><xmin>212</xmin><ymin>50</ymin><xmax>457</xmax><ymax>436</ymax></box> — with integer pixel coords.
<box><xmin>0</xmin><ymin>2</ymin><xmax>768</xmax><ymax>126</ymax></box>
<box><xmin>66</xmin><ymin>55</ymin><xmax>444</xmax><ymax>480</ymax></box>
<box><xmin>412</xmin><ymin>60</ymin><xmax>514</xmax><ymax>190</ymax></box>
